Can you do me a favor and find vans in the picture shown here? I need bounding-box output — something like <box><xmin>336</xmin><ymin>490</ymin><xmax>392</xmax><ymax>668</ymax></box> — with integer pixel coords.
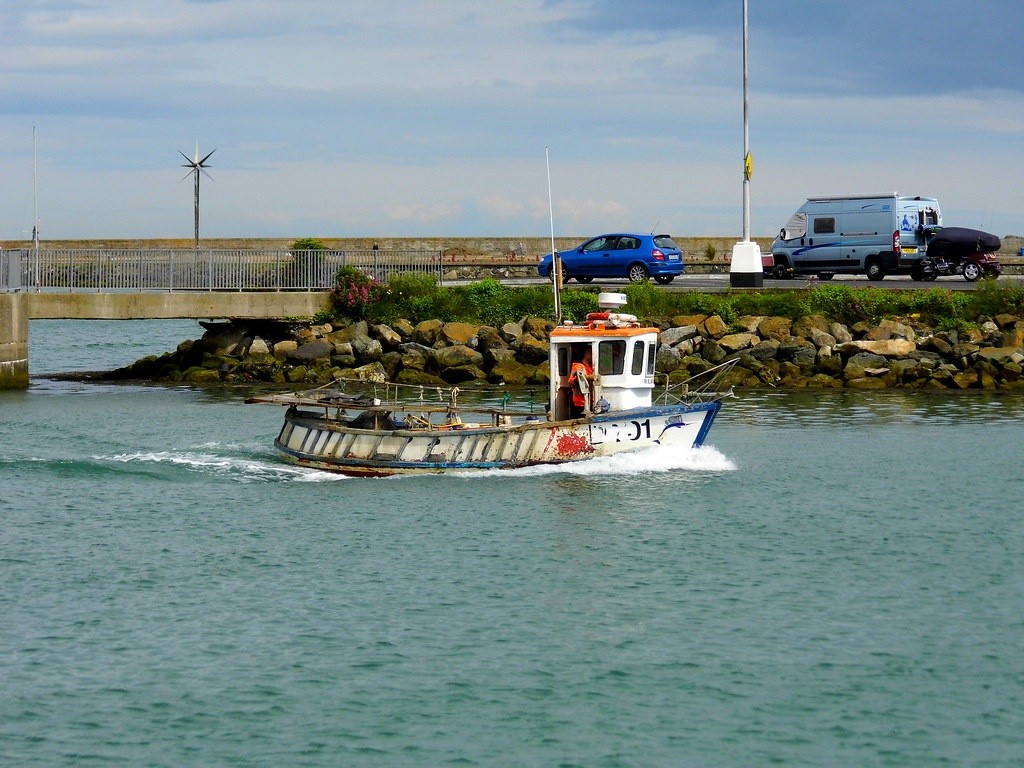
<box><xmin>769</xmin><ymin>191</ymin><xmax>943</xmax><ymax>281</ymax></box>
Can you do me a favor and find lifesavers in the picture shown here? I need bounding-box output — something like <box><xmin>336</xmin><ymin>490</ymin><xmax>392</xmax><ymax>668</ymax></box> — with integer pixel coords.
<box><xmin>586</xmin><ymin>313</ymin><xmax>640</xmax><ymax>327</ymax></box>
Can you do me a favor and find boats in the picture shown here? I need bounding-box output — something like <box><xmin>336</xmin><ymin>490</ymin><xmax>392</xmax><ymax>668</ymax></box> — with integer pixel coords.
<box><xmin>272</xmin><ymin>292</ymin><xmax>742</xmax><ymax>478</ymax></box>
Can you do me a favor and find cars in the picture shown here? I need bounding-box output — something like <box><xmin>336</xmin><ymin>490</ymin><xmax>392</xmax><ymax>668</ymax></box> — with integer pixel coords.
<box><xmin>536</xmin><ymin>232</ymin><xmax>686</xmax><ymax>284</ymax></box>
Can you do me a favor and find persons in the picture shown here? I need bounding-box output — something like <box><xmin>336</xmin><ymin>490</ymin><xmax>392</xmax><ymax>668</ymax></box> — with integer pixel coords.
<box><xmin>567</xmin><ymin>346</ymin><xmax>595</xmax><ymax>420</ymax></box>
<box><xmin>371</xmin><ymin>242</ymin><xmax>379</xmax><ymax>258</ymax></box>
